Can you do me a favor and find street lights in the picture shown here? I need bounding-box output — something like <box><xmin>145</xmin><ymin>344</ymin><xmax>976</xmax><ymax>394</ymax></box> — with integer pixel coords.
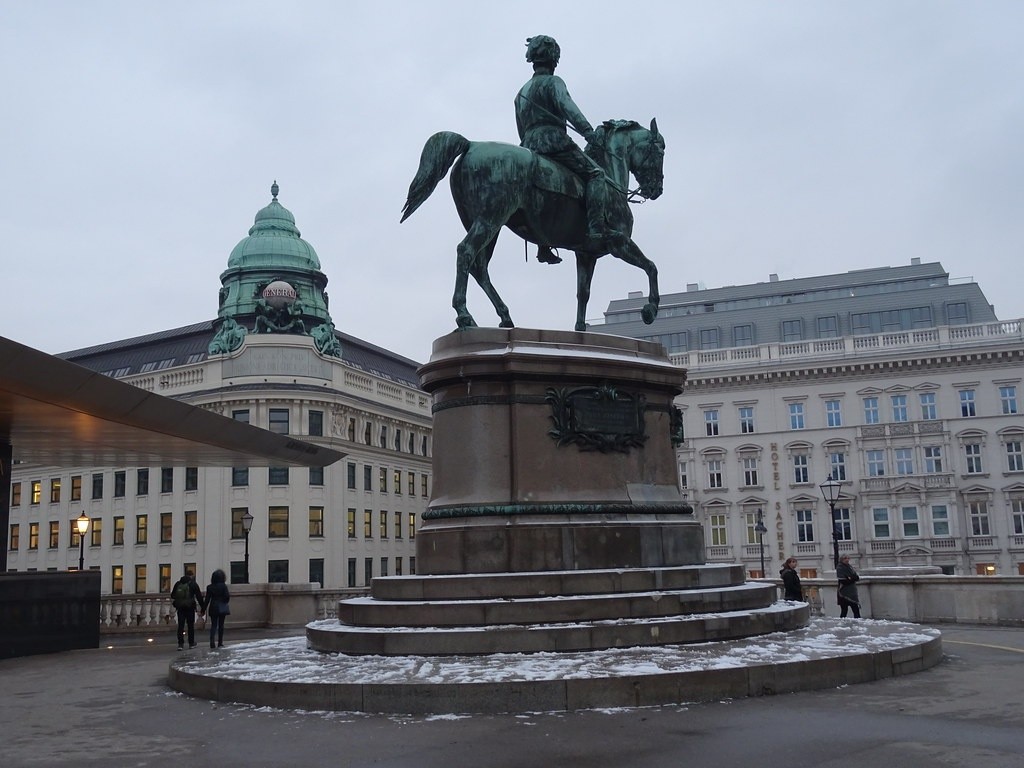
<box><xmin>755</xmin><ymin>508</ymin><xmax>768</xmax><ymax>578</ymax></box>
<box><xmin>819</xmin><ymin>473</ymin><xmax>843</xmax><ymax>569</ymax></box>
<box><xmin>241</xmin><ymin>510</ymin><xmax>254</xmax><ymax>583</ymax></box>
<box><xmin>76</xmin><ymin>510</ymin><xmax>90</xmax><ymax>570</ymax></box>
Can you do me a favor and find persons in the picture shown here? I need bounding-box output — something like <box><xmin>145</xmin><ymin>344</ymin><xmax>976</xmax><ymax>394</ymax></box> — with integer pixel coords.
<box><xmin>779</xmin><ymin>558</ymin><xmax>803</xmax><ymax>602</ymax></box>
<box><xmin>198</xmin><ymin>569</ymin><xmax>231</xmax><ymax>651</ymax></box>
<box><xmin>514</xmin><ymin>35</ymin><xmax>623</xmax><ymax>264</ymax></box>
<box><xmin>171</xmin><ymin>570</ymin><xmax>205</xmax><ymax>651</ymax></box>
<box><xmin>836</xmin><ymin>553</ymin><xmax>861</xmax><ymax>618</ymax></box>
<box><xmin>212</xmin><ymin>311</ymin><xmax>237</xmax><ymax>358</ymax></box>
<box><xmin>317</xmin><ymin>315</ymin><xmax>334</xmax><ymax>356</ymax></box>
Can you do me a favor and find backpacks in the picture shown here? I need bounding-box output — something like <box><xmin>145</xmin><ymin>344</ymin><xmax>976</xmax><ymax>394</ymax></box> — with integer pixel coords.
<box><xmin>172</xmin><ymin>580</ymin><xmax>193</xmax><ymax>611</ymax></box>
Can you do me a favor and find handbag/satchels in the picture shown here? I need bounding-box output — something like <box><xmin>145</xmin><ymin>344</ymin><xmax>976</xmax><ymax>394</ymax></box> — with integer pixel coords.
<box><xmin>217</xmin><ymin>602</ymin><xmax>231</xmax><ymax>615</ymax></box>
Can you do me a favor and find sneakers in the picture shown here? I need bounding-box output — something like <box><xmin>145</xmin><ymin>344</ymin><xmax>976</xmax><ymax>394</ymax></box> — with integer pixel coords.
<box><xmin>178</xmin><ymin>644</ymin><xmax>184</xmax><ymax>650</ymax></box>
<box><xmin>189</xmin><ymin>643</ymin><xmax>198</xmax><ymax>649</ymax></box>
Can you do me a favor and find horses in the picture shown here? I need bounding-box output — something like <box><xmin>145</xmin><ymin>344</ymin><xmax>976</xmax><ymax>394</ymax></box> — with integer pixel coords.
<box><xmin>399</xmin><ymin>116</ymin><xmax>666</xmax><ymax>330</ymax></box>
